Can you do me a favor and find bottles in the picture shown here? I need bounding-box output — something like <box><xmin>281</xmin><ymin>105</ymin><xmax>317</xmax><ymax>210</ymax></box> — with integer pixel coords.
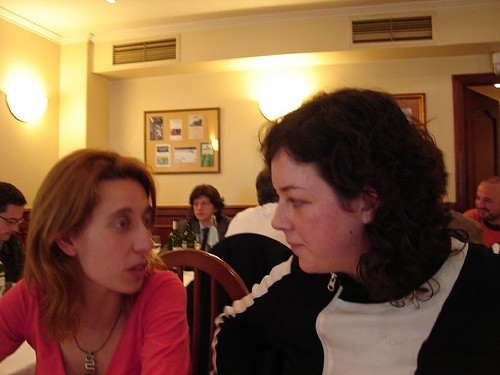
<box><xmin>0</xmin><ymin>261</ymin><xmax>5</xmax><ymax>294</ymax></box>
<box><xmin>167</xmin><ymin>219</ymin><xmax>183</xmax><ymax>269</ymax></box>
<box><xmin>181</xmin><ymin>219</ymin><xmax>196</xmax><ymax>250</ymax></box>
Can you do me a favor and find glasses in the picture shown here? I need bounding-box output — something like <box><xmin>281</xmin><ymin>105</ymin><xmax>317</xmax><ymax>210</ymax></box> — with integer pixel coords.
<box><xmin>0</xmin><ymin>216</ymin><xmax>25</xmax><ymax>225</ymax></box>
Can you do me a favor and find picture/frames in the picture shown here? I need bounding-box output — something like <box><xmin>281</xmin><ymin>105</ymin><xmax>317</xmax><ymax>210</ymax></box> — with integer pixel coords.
<box><xmin>392</xmin><ymin>93</ymin><xmax>425</xmax><ymax>125</ymax></box>
<box><xmin>143</xmin><ymin>107</ymin><xmax>220</xmax><ymax>175</ymax></box>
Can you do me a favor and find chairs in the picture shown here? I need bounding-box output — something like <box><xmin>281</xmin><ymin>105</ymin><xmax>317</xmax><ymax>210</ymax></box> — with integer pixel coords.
<box><xmin>159</xmin><ymin>249</ymin><xmax>248</xmax><ymax>375</ymax></box>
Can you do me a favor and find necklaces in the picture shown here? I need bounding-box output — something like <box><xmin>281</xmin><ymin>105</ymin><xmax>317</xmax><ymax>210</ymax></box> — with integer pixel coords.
<box><xmin>69</xmin><ymin>303</ymin><xmax>119</xmax><ymax>375</ymax></box>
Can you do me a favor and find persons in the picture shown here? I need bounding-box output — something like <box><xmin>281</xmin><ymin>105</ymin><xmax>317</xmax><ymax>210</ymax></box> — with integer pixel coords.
<box><xmin>0</xmin><ymin>170</ymin><xmax>499</xmax><ymax>284</ymax></box>
<box><xmin>205</xmin><ymin>89</ymin><xmax>500</xmax><ymax>375</ymax></box>
<box><xmin>1</xmin><ymin>149</ymin><xmax>190</xmax><ymax>375</ymax></box>
